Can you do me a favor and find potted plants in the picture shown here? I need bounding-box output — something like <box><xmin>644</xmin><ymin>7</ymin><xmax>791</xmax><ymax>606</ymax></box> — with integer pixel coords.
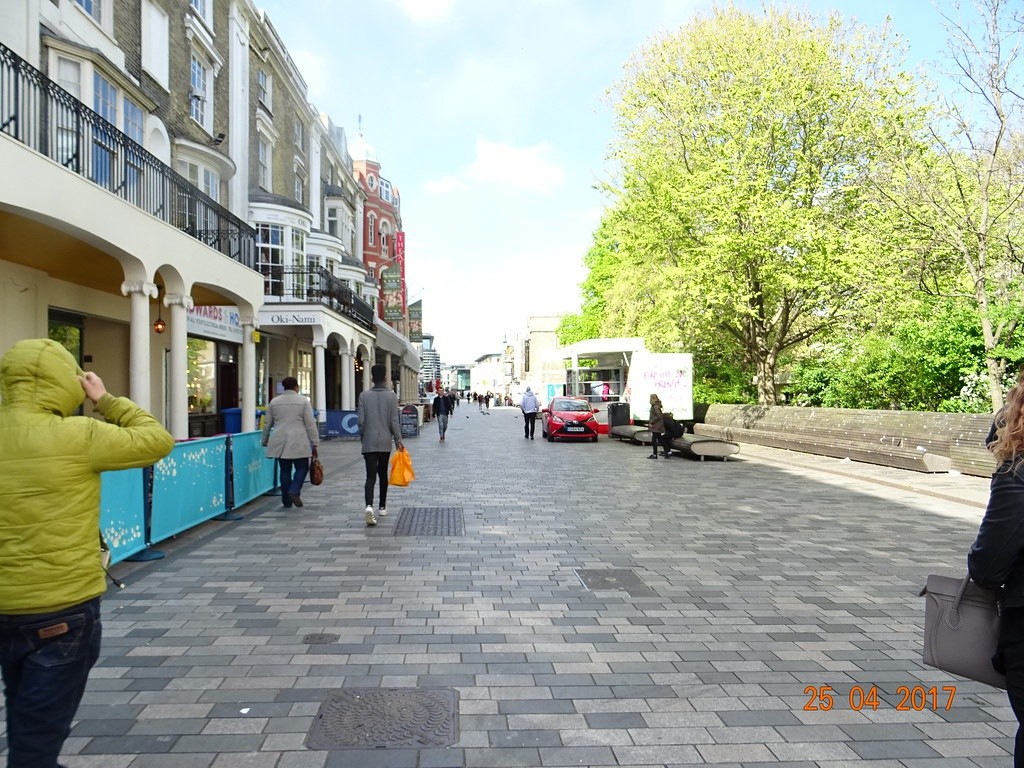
<box><xmin>188</xmin><ymin>395</ymin><xmax>199</xmax><ymax>414</ymax></box>
<box><xmin>200</xmin><ymin>396</ymin><xmax>213</xmax><ymax>414</ymax></box>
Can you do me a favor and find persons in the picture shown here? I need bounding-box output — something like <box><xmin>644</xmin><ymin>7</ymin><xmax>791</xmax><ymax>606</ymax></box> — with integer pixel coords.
<box><xmin>504</xmin><ymin>393</ymin><xmax>508</xmax><ymax>406</ymax></box>
<box><xmin>432</xmin><ymin>387</ymin><xmax>453</xmax><ymax>443</ymax></box>
<box><xmin>658</xmin><ymin>413</ymin><xmax>684</xmax><ymax>456</ymax></box>
<box><xmin>0</xmin><ymin>338</ymin><xmax>174</xmax><ymax>768</ymax></box>
<box><xmin>466</xmin><ymin>391</ymin><xmax>478</xmax><ymax>404</ymax></box>
<box><xmin>967</xmin><ymin>365</ymin><xmax>1024</xmax><ymax>768</ymax></box>
<box><xmin>478</xmin><ymin>394</ymin><xmax>483</xmax><ymax>410</ymax></box>
<box><xmin>261</xmin><ymin>376</ymin><xmax>318</xmax><ymax>507</ymax></box>
<box><xmin>646</xmin><ymin>394</ymin><xmax>673</xmax><ymax>458</ymax></box>
<box><xmin>444</xmin><ymin>388</ymin><xmax>464</xmax><ymax>416</ymax></box>
<box><xmin>484</xmin><ymin>392</ymin><xmax>491</xmax><ymax>408</ymax></box>
<box><xmin>519</xmin><ymin>386</ymin><xmax>539</xmax><ymax>440</ymax></box>
<box><xmin>357</xmin><ymin>363</ymin><xmax>404</xmax><ymax>525</ymax></box>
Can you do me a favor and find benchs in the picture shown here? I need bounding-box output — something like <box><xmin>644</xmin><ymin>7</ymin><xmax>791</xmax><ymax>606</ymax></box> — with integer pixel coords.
<box><xmin>612</xmin><ymin>426</ymin><xmax>652</xmax><ymax>446</ymax></box>
<box><xmin>671</xmin><ymin>431</ymin><xmax>742</xmax><ymax>462</ymax></box>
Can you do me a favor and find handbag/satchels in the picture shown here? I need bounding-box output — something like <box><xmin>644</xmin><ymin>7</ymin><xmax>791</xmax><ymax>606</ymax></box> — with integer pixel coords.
<box><xmin>310</xmin><ymin>448</ymin><xmax>323</xmax><ymax>485</ymax></box>
<box><xmin>918</xmin><ymin>573</ymin><xmax>1007</xmax><ymax>691</ymax></box>
<box><xmin>388</xmin><ymin>447</ymin><xmax>415</xmax><ymax>486</ymax></box>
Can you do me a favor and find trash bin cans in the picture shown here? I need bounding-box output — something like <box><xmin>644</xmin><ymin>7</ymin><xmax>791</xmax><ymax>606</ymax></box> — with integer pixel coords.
<box><xmin>607</xmin><ymin>402</ymin><xmax>631</xmax><ymax>439</ymax></box>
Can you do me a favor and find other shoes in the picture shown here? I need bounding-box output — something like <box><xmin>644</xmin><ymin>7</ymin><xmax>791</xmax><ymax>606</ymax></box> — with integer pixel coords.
<box><xmin>440</xmin><ymin>435</ymin><xmax>445</xmax><ymax>440</ymax></box>
<box><xmin>378</xmin><ymin>505</ymin><xmax>387</xmax><ymax>516</ymax></box>
<box><xmin>665</xmin><ymin>452</ymin><xmax>672</xmax><ymax>458</ymax></box>
<box><xmin>650</xmin><ymin>455</ymin><xmax>656</xmax><ymax>459</ymax></box>
<box><xmin>531</xmin><ymin>435</ymin><xmax>534</xmax><ymax>439</ymax></box>
<box><xmin>289</xmin><ymin>493</ymin><xmax>303</xmax><ymax>507</ymax></box>
<box><xmin>661</xmin><ymin>452</ymin><xmax>666</xmax><ymax>456</ymax></box>
<box><xmin>283</xmin><ymin>501</ymin><xmax>292</xmax><ymax>507</ymax></box>
<box><xmin>365</xmin><ymin>505</ymin><xmax>376</xmax><ymax>524</ymax></box>
<box><xmin>525</xmin><ymin>435</ymin><xmax>529</xmax><ymax>438</ymax></box>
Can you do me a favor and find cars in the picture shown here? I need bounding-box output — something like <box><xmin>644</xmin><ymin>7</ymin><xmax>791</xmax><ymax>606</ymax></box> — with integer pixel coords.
<box><xmin>540</xmin><ymin>397</ymin><xmax>601</xmax><ymax>443</ymax></box>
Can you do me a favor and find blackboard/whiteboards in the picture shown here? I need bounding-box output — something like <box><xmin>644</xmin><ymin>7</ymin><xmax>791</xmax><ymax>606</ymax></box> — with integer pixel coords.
<box><xmin>401</xmin><ymin>404</ymin><xmax>419</xmax><ymax>437</ymax></box>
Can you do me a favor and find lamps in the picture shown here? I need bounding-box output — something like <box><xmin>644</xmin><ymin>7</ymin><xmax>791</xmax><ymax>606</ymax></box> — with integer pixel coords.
<box><xmin>153</xmin><ymin>284</ymin><xmax>165</xmax><ymax>335</ymax></box>
